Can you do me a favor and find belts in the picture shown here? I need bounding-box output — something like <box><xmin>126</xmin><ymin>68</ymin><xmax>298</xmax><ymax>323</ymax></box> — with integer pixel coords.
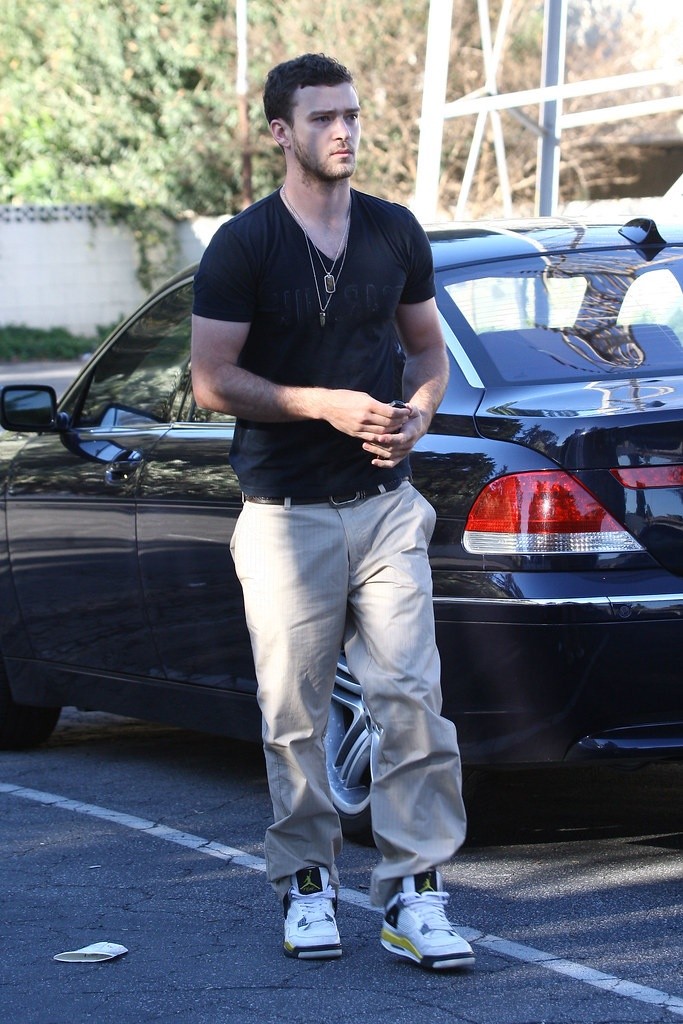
<box><xmin>245</xmin><ymin>477</ymin><xmax>402</xmax><ymax>508</ymax></box>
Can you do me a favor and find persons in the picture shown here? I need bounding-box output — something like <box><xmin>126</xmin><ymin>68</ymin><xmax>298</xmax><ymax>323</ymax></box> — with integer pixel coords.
<box><xmin>191</xmin><ymin>52</ymin><xmax>477</xmax><ymax>969</ymax></box>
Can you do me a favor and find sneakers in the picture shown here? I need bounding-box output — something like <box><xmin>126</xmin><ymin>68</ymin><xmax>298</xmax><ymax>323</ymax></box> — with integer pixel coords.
<box><xmin>380</xmin><ymin>871</ymin><xmax>476</xmax><ymax>968</ymax></box>
<box><xmin>283</xmin><ymin>865</ymin><xmax>342</xmax><ymax>957</ymax></box>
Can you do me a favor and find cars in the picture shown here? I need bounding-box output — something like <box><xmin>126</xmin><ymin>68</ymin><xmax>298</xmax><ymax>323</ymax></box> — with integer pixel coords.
<box><xmin>0</xmin><ymin>216</ymin><xmax>683</xmax><ymax>845</ymax></box>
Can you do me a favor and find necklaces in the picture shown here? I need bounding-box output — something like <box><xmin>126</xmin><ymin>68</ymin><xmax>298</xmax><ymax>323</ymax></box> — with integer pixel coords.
<box><xmin>283</xmin><ymin>184</ymin><xmax>351</xmax><ymax>328</ymax></box>
